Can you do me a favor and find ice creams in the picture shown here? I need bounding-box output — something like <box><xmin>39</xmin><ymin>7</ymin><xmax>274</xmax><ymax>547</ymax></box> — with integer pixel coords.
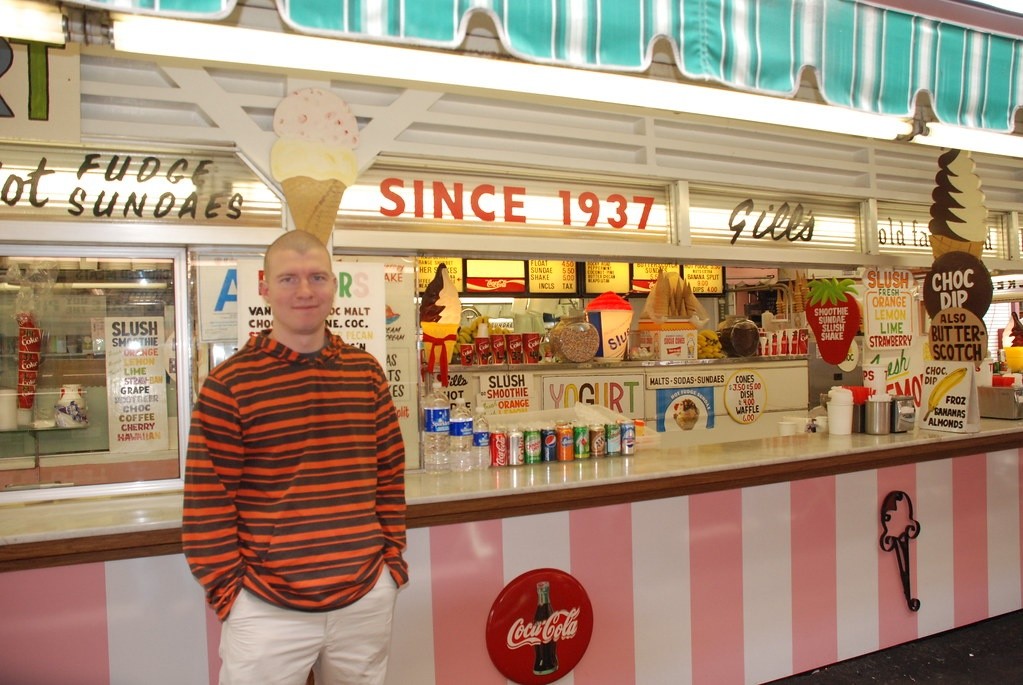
<box><xmin>418</xmin><ymin>263</ymin><xmax>463</xmax><ymax>366</ymax></box>
<box><xmin>928</xmin><ymin>149</ymin><xmax>990</xmax><ymax>261</ymax></box>
<box><xmin>270</xmin><ymin>88</ymin><xmax>359</xmax><ymax>247</ymax></box>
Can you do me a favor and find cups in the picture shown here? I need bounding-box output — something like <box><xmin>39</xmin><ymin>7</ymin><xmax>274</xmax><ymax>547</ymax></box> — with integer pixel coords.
<box><xmin>458</xmin><ymin>332</ymin><xmax>540</xmax><ymax>365</ymax></box>
<box><xmin>759</xmin><ymin>329</ymin><xmax>809</xmax><ymax>356</ymax></box>
<box><xmin>825</xmin><ymin>403</ymin><xmax>853</xmax><ymax>435</ymax></box>
<box><xmin>1004</xmin><ymin>346</ymin><xmax>1023</xmax><ymax>371</ymax></box>
<box><xmin>583</xmin><ymin>310</ymin><xmax>634</xmax><ymax>362</ymax></box>
<box><xmin>0</xmin><ymin>313</ymin><xmax>57</xmax><ymax>430</ymax></box>
<box><xmin>868</xmin><ymin>401</ymin><xmax>890</xmax><ymax>434</ymax></box>
<box><xmin>862</xmin><ymin>364</ymin><xmax>886</xmax><ymax>396</ymax></box>
<box><xmin>974</xmin><ymin>358</ymin><xmax>993</xmax><ymax>388</ymax></box>
<box><xmin>627</xmin><ymin>330</ymin><xmax>655</xmax><ymax>360</ymax></box>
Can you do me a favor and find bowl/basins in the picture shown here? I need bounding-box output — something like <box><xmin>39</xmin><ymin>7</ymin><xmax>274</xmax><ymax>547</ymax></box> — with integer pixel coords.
<box><xmin>676</xmin><ymin>416</ymin><xmax>698</xmax><ymax>430</ymax></box>
<box><xmin>779</xmin><ymin>416</ymin><xmax>828</xmax><ymax>437</ymax></box>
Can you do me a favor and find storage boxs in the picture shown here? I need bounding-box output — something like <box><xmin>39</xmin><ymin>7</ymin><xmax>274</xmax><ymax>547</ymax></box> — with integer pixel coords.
<box><xmin>638</xmin><ymin>317</ymin><xmax>698</xmax><ymax>360</ymax></box>
<box><xmin>979</xmin><ymin>386</ymin><xmax>1023</xmax><ymax>419</ymax></box>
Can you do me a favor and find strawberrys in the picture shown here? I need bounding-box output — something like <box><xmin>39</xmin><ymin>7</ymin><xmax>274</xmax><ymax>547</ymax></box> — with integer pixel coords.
<box><xmin>805</xmin><ymin>277</ymin><xmax>861</xmax><ymax>365</ymax></box>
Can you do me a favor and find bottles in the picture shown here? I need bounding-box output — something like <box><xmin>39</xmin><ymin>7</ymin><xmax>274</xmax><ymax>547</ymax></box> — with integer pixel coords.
<box><xmin>762</xmin><ymin>310</ymin><xmax>772</xmax><ymax>328</ymax></box>
<box><xmin>551</xmin><ymin>312</ymin><xmax>599</xmax><ymax>363</ymax></box>
<box><xmin>635</xmin><ymin>421</ymin><xmax>647</xmax><ymax>438</ymax></box>
<box><xmin>423</xmin><ymin>383</ymin><xmax>451</xmax><ymax>472</ymax></box>
<box><xmin>718</xmin><ymin>314</ymin><xmax>759</xmax><ymax>357</ymax></box>
<box><xmin>470</xmin><ymin>405</ymin><xmax>491</xmax><ymax>471</ymax></box>
<box><xmin>477</xmin><ymin>319</ymin><xmax>488</xmax><ymax>338</ymax></box>
<box><xmin>451</xmin><ymin>397</ymin><xmax>472</xmax><ymax>470</ymax></box>
<box><xmin>533</xmin><ymin>582</ymin><xmax>559</xmax><ymax>675</ymax></box>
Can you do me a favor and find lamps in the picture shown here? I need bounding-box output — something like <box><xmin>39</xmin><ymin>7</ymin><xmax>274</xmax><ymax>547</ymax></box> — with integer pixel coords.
<box><xmin>1</xmin><ymin>1</ymin><xmax>1023</xmax><ymax>158</ymax></box>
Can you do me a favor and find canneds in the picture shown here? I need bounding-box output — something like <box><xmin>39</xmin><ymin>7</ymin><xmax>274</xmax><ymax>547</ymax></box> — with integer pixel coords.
<box><xmin>490</xmin><ymin>420</ymin><xmax>636</xmax><ymax>467</ymax></box>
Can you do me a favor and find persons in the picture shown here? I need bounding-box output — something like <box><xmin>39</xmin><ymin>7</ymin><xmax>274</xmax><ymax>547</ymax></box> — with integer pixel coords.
<box><xmin>181</xmin><ymin>231</ymin><xmax>410</xmax><ymax>685</ymax></box>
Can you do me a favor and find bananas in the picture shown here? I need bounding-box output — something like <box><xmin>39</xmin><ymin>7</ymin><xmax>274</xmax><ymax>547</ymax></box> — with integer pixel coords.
<box><xmin>697</xmin><ymin>330</ymin><xmax>726</xmax><ymax>359</ymax></box>
<box><xmin>453</xmin><ymin>316</ymin><xmax>511</xmax><ymax>354</ymax></box>
